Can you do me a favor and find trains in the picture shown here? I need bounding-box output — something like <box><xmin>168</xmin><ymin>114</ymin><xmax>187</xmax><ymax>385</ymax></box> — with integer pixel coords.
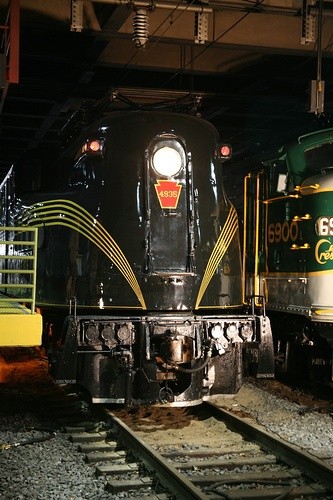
<box><xmin>224</xmin><ymin>126</ymin><xmax>333</xmax><ymax>383</ymax></box>
<box><xmin>0</xmin><ymin>85</ymin><xmax>275</xmax><ymax>416</ymax></box>
